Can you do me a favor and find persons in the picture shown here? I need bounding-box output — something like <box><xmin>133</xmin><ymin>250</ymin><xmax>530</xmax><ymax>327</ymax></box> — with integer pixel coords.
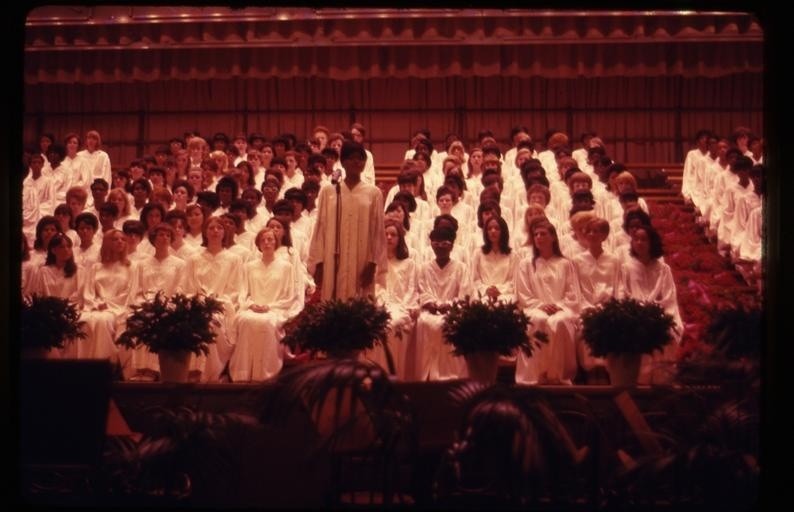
<box><xmin>623</xmin><ymin>226</ymin><xmax>684</xmax><ymax>385</ymax></box>
<box><xmin>169</xmin><ymin>179</ymin><xmax>194</xmax><ymax>213</ymax></box>
<box><xmin>384</xmin><ymin>200</ymin><xmax>418</xmax><ymax>247</ymax></box>
<box><xmin>572</xmin><ymin>132</ymin><xmax>595</xmax><ymax>161</ymax></box>
<box><xmin>605</xmin><ymin>171</ymin><xmax>650</xmax><ymax>218</ymax></box>
<box><xmin>716</xmin><ymin>156</ymin><xmax>753</xmax><ymax>262</ymax></box>
<box><xmin>253</xmin><ymin>179</ymin><xmax>281</xmax><ymax>216</ymax></box>
<box><xmin>517</xmin><ymin>176</ymin><xmax>559</xmax><ymax>221</ymax></box>
<box><xmin>86</xmin><ymin>176</ymin><xmax>110</xmax><ymax>226</ymax></box>
<box><xmin>397</xmin><ymin>172</ymin><xmax>430</xmax><ymax>219</ymax></box>
<box><xmin>697</xmin><ymin>140</ymin><xmax>736</xmax><ymax>227</ymax></box>
<box><xmin>425</xmin><ymin>186</ymin><xmax>465</xmax><ymax>230</ymax></box>
<box><xmin>166</xmin><ymin>209</ymin><xmax>198</xmax><ymax>260</ymax></box>
<box><xmin>255</xmin><ymin>216</ymin><xmax>300</xmax><ymax>262</ymax></box>
<box><xmin>188</xmin><ymin>137</ymin><xmax>210</xmax><ymax>168</ymax></box>
<box><xmin>24</xmin><ymin>155</ymin><xmax>57</xmax><ymax>215</ymax></box>
<box><xmin>308</xmin><ymin>136</ymin><xmax>321</xmax><ymax>152</ymax></box>
<box><xmin>560</xmin><ymin>189</ymin><xmax>596</xmax><ymax>236</ymax></box>
<box><xmin>558</xmin><ymin>207</ymin><xmax>604</xmax><ymax>253</ymax></box>
<box><xmin>22</xmin><ymin>230</ymin><xmax>31</xmax><ymax>261</ymax></box>
<box><xmin>539</xmin><ymin>129</ymin><xmax>569</xmax><ymax>156</ymax></box>
<box><xmin>313</xmin><ymin>127</ymin><xmax>330</xmax><ymax>147</ymax></box>
<box><xmin>149</xmin><ymin>165</ymin><xmax>167</xmax><ymax>192</ymax></box>
<box><xmin>745</xmin><ymin>136</ymin><xmax>765</xmax><ymax>165</ymax></box>
<box><xmin>246</xmin><ymin>149</ymin><xmax>266</xmax><ymax>190</ymax></box>
<box><xmin>514</xmin><ymin>149</ymin><xmax>531</xmax><ymax>168</ymax></box>
<box><xmin>690</xmin><ymin>135</ymin><xmax>722</xmax><ymax>215</ymax></box>
<box><xmin>271</xmin><ymin>135</ymin><xmax>289</xmax><ymax>159</ymax></box>
<box><xmin>514</xmin><ymin>184</ymin><xmax>562</xmax><ymax>234</ymax></box>
<box><xmin>76</xmin><ymin>226</ymin><xmax>134</xmax><ymax>359</ymax></box>
<box><xmin>681</xmin><ymin>129</ymin><xmax>712</xmax><ymax>206</ymax></box>
<box><xmin>122</xmin><ymin>219</ymin><xmax>151</xmax><ymax>265</ymax></box>
<box><xmin>176</xmin><ymin>149</ymin><xmax>191</xmax><ymax>181</ymax></box>
<box><xmin>613</xmin><ymin>210</ymin><xmax>665</xmax><ymax>264</ymax></box>
<box><xmin>107</xmin><ymin>188</ymin><xmax>140</xmax><ymax>230</ymax></box>
<box><xmin>481</xmin><ymin>187</ymin><xmax>501</xmax><ymax>208</ymax></box>
<box><xmin>129</xmin><ymin>158</ymin><xmax>146</xmax><ymax>186</ymax></box>
<box><xmin>403</xmin><ymin>127</ymin><xmax>431</xmax><ymax>160</ymax></box>
<box><xmin>284</xmin><ymin>187</ymin><xmax>313</xmax><ymax>240</ymax></box>
<box><xmin>598</xmin><ymin>164</ymin><xmax>628</xmax><ymax>205</ymax></box>
<box><xmin>417</xmin><ymin>226</ymin><xmax>469</xmax><ymax>381</ymax></box>
<box><xmin>590</xmin><ymin>136</ymin><xmax>603</xmax><ymax>148</ymax></box>
<box><xmin>393</xmin><ymin>190</ymin><xmax>417</xmax><ymax>214</ymax></box>
<box><xmin>479</xmin><ymin>128</ymin><xmax>493</xmax><ymax>144</ymax></box>
<box><xmin>272</xmin><ymin>200</ymin><xmax>308</xmax><ymax>261</ymax></box>
<box><xmin>412</xmin><ymin>152</ymin><xmax>432</xmax><ymax>172</ymax></box>
<box><xmin>516</xmin><ymin>163</ymin><xmax>554</xmax><ymax>207</ymax></box>
<box><xmin>213</xmin><ymin>132</ymin><xmax>229</xmax><ymax>152</ymax></box>
<box><xmin>552</xmin><ymin>159</ymin><xmax>578</xmax><ymax>189</ymax></box>
<box><xmin>415</xmin><ymin>139</ymin><xmax>433</xmax><ymax>157</ymax></box>
<box><xmin>309</xmin><ymin>140</ymin><xmax>385</xmax><ymax>303</ymax></box>
<box><xmin>424</xmin><ymin>214</ymin><xmax>469</xmax><ymax>262</ymax></box>
<box><xmin>187</xmin><ymin>167</ymin><xmax>206</xmax><ymax>206</ymax></box>
<box><xmin>66</xmin><ymin>186</ymin><xmax>88</xmax><ymax>228</ymax></box>
<box><xmin>592</xmin><ymin>157</ymin><xmax>612</xmax><ymax>192</ymax></box>
<box><xmin>468</xmin><ymin>201</ymin><xmax>502</xmax><ymax>251</ymax></box>
<box><xmin>196</xmin><ymin>190</ymin><xmax>219</xmax><ymax>217</ymax></box>
<box><xmin>236</xmin><ymin>160</ymin><xmax>255</xmax><ymax>190</ymax></box>
<box><xmin>384</xmin><ymin>159</ymin><xmax>417</xmax><ymax>212</ymax></box>
<box><xmin>510</xmin><ymin>205</ymin><xmax>552</xmax><ymax>251</ymax></box>
<box><xmin>546</xmin><ymin>146</ymin><xmax>575</xmax><ymax>175</ymax></box>
<box><xmin>446</xmin><ymin>166</ymin><xmax>474</xmax><ymax>205</ymax></box>
<box><xmin>483</xmin><ymin>175</ymin><xmax>505</xmax><ymax>194</ymax></box>
<box><xmin>706</xmin><ymin>148</ymin><xmax>742</xmax><ymax>243</ymax></box>
<box><xmin>442</xmin><ymin>157</ymin><xmax>461</xmax><ymax>175</ymax></box>
<box><xmin>61</xmin><ymin>132</ymin><xmax>91</xmax><ymax>189</ymax></box>
<box><xmin>270</xmin><ymin>156</ymin><xmax>292</xmax><ymax>192</ymax></box>
<box><xmin>54</xmin><ymin>203</ymin><xmax>80</xmax><ymax>247</ymax></box>
<box><xmin>584</xmin><ymin>148</ymin><xmax>605</xmax><ymax>177</ymax></box>
<box><xmin>154</xmin><ymin>147</ymin><xmax>167</xmax><ymax>166</ymax></box>
<box><xmin>745</xmin><ymin>183</ymin><xmax>764</xmax><ymax>260</ymax></box>
<box><xmin>295</xmin><ymin>144</ymin><xmax>311</xmax><ymax>170</ymax></box>
<box><xmin>570</xmin><ymin>218</ymin><xmax>621</xmax><ymax>385</ymax></box>
<box><xmin>209</xmin><ymin>150</ymin><xmax>227</xmax><ymax>182</ymax></box>
<box><xmin>76</xmin><ymin>130</ymin><xmax>112</xmax><ymax>200</ymax></box>
<box><xmin>340</xmin><ymin>129</ymin><xmax>351</xmax><ymax>141</ymax></box>
<box><xmin>40</xmin><ymin>142</ymin><xmax>73</xmax><ymax>203</ymax></box>
<box><xmin>320</xmin><ymin>147</ymin><xmax>339</xmax><ymax>182</ymax></box>
<box><xmin>308</xmin><ymin>153</ymin><xmax>332</xmax><ymax>188</ymax></box>
<box><xmin>736</xmin><ymin>163</ymin><xmax>764</xmax><ymax>295</ymax></box>
<box><xmin>22</xmin><ymin>158</ymin><xmax>40</xmax><ymax>248</ymax></box>
<box><xmin>264</xmin><ymin>168</ymin><xmax>285</xmax><ymax>199</ymax></box>
<box><xmin>517</xmin><ymin>142</ymin><xmax>535</xmax><ymax>156</ymax></box>
<box><xmin>431</xmin><ymin>175</ymin><xmax>475</xmax><ymax>220</ymax></box>
<box><xmin>200</xmin><ymin>157</ymin><xmax>218</xmax><ymax>194</ymax></box>
<box><xmin>374</xmin><ymin>219</ymin><xmax>418</xmax><ymax>379</ymax></box>
<box><xmin>242</xmin><ymin>189</ymin><xmax>270</xmax><ymax>233</ymax></box>
<box><xmin>609</xmin><ymin>191</ymin><xmax>646</xmax><ymax>236</ymax></box>
<box><xmin>183</xmin><ymin>203</ymin><xmax>205</xmax><ymax>244</ymax></box>
<box><xmin>283</xmin><ymin>133</ymin><xmax>296</xmax><ymax>150</ymax></box>
<box><xmin>248</xmin><ymin>132</ymin><xmax>266</xmax><ymax>149</ymax></box>
<box><xmin>511</xmin><ymin>126</ymin><xmax>530</xmax><ymax>146</ymax></box>
<box><xmin>553</xmin><ymin>167</ymin><xmax>584</xmax><ymax>207</ymax></box>
<box><xmin>136</xmin><ymin>202</ymin><xmax>167</xmax><ymax>254</ymax></box>
<box><xmin>183</xmin><ymin>128</ymin><xmax>200</xmax><ymax>146</ymax></box>
<box><xmin>123</xmin><ymin>222</ymin><xmax>187</xmax><ymax>382</ymax></box>
<box><xmin>22</xmin><ymin>232</ymin><xmax>89</xmax><ymax>359</ymax></box>
<box><xmin>516</xmin><ymin>223</ymin><xmax>582</xmax><ymax>385</ymax></box>
<box><xmin>481</xmin><ymin>154</ymin><xmax>501</xmax><ymax>172</ymax></box>
<box><xmin>28</xmin><ymin>216</ymin><xmax>63</xmax><ymax>265</ymax></box>
<box><xmin>470</xmin><ymin>215</ymin><xmax>521</xmax><ymax>307</ymax></box>
<box><xmin>164</xmin><ymin>136</ymin><xmax>185</xmax><ymax>155</ymax></box>
<box><xmin>301</xmin><ymin>179</ymin><xmax>321</xmax><ymax>218</ymax></box>
<box><xmin>483</xmin><ymin>146</ymin><xmax>501</xmax><ymax>158</ymax></box>
<box><xmin>303</xmin><ymin>167</ymin><xmax>330</xmax><ymax>189</ymax></box>
<box><xmin>260</xmin><ymin>143</ymin><xmax>276</xmax><ymax>170</ymax></box>
<box><xmin>438</xmin><ymin>133</ymin><xmax>470</xmax><ymax>158</ymax></box>
<box><xmin>232</xmin><ymin>135</ymin><xmax>248</xmax><ymax>167</ymax></box>
<box><xmin>283</xmin><ymin>150</ymin><xmax>305</xmax><ymax>189</ymax></box>
<box><xmin>129</xmin><ymin>178</ymin><xmax>152</xmax><ymax>219</ymax></box>
<box><xmin>327</xmin><ymin>132</ymin><xmax>347</xmax><ymax>179</ymax></box>
<box><xmin>150</xmin><ymin>188</ymin><xmax>172</xmax><ymax>212</ymax></box>
<box><xmin>92</xmin><ymin>200</ymin><xmax>117</xmax><ymax>244</ymax></box>
<box><xmin>220</xmin><ymin>213</ymin><xmax>254</xmax><ymax>262</ymax></box>
<box><xmin>143</xmin><ymin>154</ymin><xmax>156</xmax><ymax>170</ymax></box>
<box><xmin>196</xmin><ymin>174</ymin><xmax>197</xmax><ymax>176</ymax></box>
<box><xmin>186</xmin><ymin>216</ymin><xmax>243</xmax><ymax>379</ymax></box>
<box><xmin>558</xmin><ymin>174</ymin><xmax>604</xmax><ymax>221</ymax></box>
<box><xmin>480</xmin><ymin>137</ymin><xmax>498</xmax><ymax>148</ymax></box>
<box><xmin>22</xmin><ymin>143</ymin><xmax>33</xmax><ymax>180</ymax></box>
<box><xmin>515</xmin><ymin>217</ymin><xmax>554</xmax><ymax>260</ymax></box>
<box><xmin>230</xmin><ymin>226</ymin><xmax>294</xmax><ymax>384</ymax></box>
<box><xmin>560</xmin><ymin>212</ymin><xmax>610</xmax><ymax>259</ymax></box>
<box><xmin>21</xmin><ymin>231</ymin><xmax>32</xmax><ymax>300</ymax></box>
<box><xmin>212</xmin><ymin>177</ymin><xmax>237</xmax><ymax>214</ymax></box>
<box><xmin>73</xmin><ymin>212</ymin><xmax>101</xmax><ymax>263</ymax></box>
<box><xmin>229</xmin><ymin>198</ymin><xmax>262</xmax><ymax>252</ymax></box>
<box><xmin>224</xmin><ymin>144</ymin><xmax>239</xmax><ymax>169</ymax></box>
<box><xmin>448</xmin><ymin>140</ymin><xmax>466</xmax><ymax>163</ymax></box>
<box><xmin>730</xmin><ymin>126</ymin><xmax>758</xmax><ymax>159</ymax></box>
<box><xmin>39</xmin><ymin>132</ymin><xmax>56</xmax><ymax>165</ymax></box>
<box><xmin>465</xmin><ymin>148</ymin><xmax>483</xmax><ymax>179</ymax></box>
<box><xmin>110</xmin><ymin>168</ymin><xmax>133</xmax><ymax>195</ymax></box>
<box><xmin>164</xmin><ymin>158</ymin><xmax>179</xmax><ymax>195</ymax></box>
<box><xmin>351</xmin><ymin>123</ymin><xmax>375</xmax><ymax>186</ymax></box>
<box><xmin>407</xmin><ymin>168</ymin><xmax>429</xmax><ymax>202</ymax></box>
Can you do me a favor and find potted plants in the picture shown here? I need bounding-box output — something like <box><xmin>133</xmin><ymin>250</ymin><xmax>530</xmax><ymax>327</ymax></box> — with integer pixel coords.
<box><xmin>20</xmin><ymin>292</ymin><xmax>90</xmax><ymax>360</ymax></box>
<box><xmin>278</xmin><ymin>294</ymin><xmax>392</xmax><ymax>378</ymax></box>
<box><xmin>114</xmin><ymin>288</ymin><xmax>226</xmax><ymax>383</ymax></box>
<box><xmin>699</xmin><ymin>300</ymin><xmax>763</xmax><ymax>373</ymax></box>
<box><xmin>441</xmin><ymin>289</ymin><xmax>549</xmax><ymax>382</ymax></box>
<box><xmin>577</xmin><ymin>295</ymin><xmax>680</xmax><ymax>386</ymax></box>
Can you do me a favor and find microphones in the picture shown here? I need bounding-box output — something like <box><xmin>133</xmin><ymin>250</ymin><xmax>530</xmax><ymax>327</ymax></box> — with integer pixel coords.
<box><xmin>330</xmin><ymin>169</ymin><xmax>341</xmax><ymax>184</ymax></box>
